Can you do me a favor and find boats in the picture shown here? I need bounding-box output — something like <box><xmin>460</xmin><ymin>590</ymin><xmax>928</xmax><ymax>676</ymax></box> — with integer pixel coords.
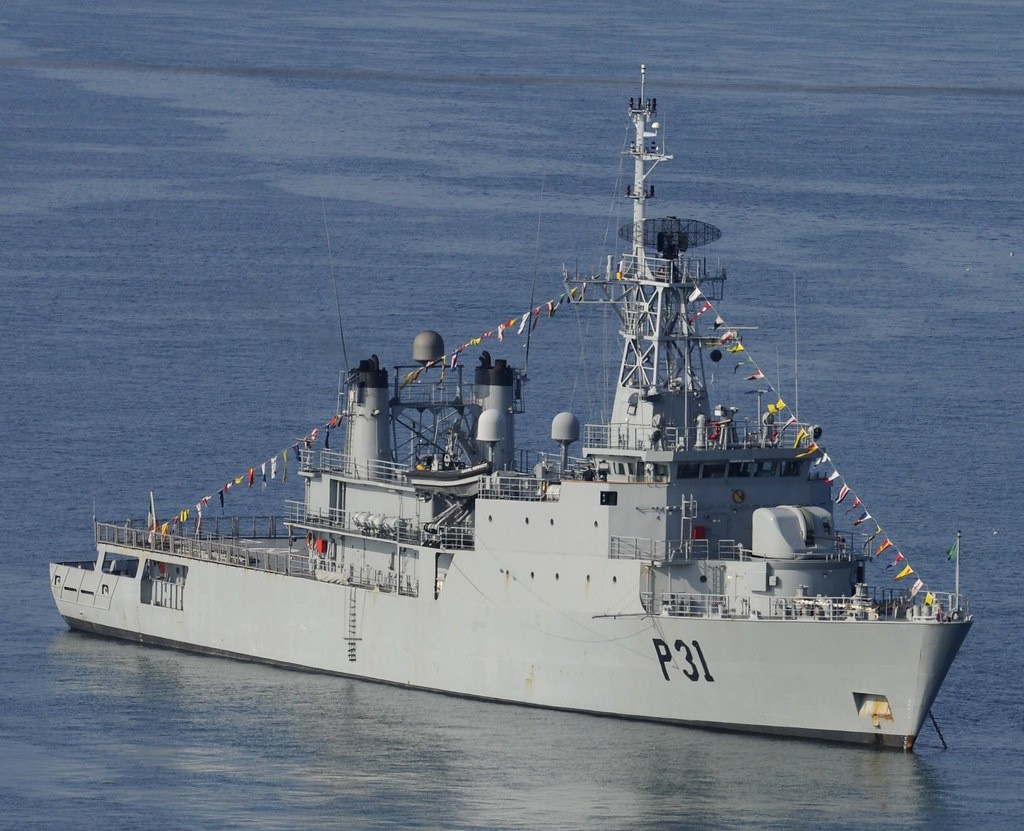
<box><xmin>49</xmin><ymin>64</ymin><xmax>973</xmax><ymax>753</ymax></box>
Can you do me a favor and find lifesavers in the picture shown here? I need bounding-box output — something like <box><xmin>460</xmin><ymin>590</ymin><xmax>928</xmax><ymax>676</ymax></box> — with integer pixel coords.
<box><xmin>306</xmin><ymin>533</ymin><xmax>316</xmax><ymax>549</ymax></box>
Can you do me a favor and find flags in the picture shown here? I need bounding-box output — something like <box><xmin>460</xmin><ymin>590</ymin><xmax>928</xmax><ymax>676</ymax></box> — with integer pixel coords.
<box><xmin>148</xmin><ymin>492</ymin><xmax>159</xmax><ymax>541</ymax></box>
<box><xmin>946</xmin><ymin>539</ymin><xmax>956</xmax><ymax>562</ymax></box>
<box><xmin>687</xmin><ymin>286</ymin><xmax>934</xmax><ymax>608</ymax></box>
<box><xmin>162</xmin><ymin>260</ymin><xmax>622</xmax><ymax>544</ymax></box>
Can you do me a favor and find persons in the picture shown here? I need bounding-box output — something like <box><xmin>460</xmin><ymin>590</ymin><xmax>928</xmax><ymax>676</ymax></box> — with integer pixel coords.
<box><xmin>570</xmin><ymin>470</ymin><xmax>575</xmax><ymax>480</ymax></box>
<box><xmin>583</xmin><ymin>465</ymin><xmax>595</xmax><ymax>481</ymax></box>
